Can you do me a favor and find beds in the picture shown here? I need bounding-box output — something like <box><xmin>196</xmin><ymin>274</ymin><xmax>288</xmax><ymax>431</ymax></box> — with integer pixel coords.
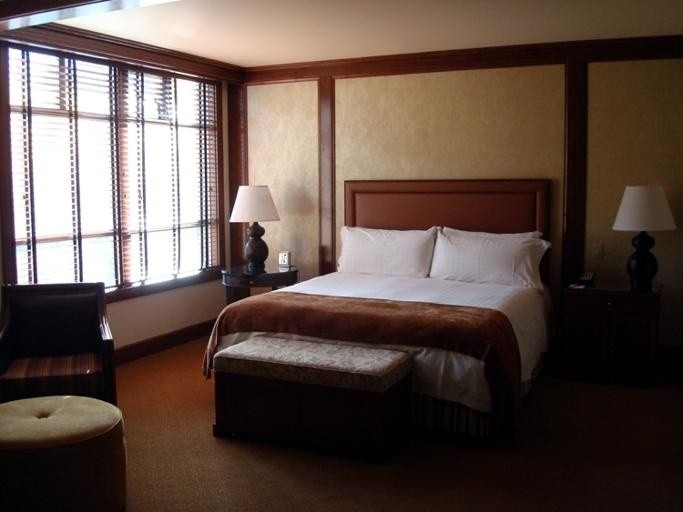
<box><xmin>201</xmin><ymin>179</ymin><xmax>555</xmax><ymax>452</ymax></box>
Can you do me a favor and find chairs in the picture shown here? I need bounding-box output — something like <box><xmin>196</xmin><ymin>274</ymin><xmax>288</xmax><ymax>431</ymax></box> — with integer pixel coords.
<box><xmin>0</xmin><ymin>283</ymin><xmax>117</xmax><ymax>407</ymax></box>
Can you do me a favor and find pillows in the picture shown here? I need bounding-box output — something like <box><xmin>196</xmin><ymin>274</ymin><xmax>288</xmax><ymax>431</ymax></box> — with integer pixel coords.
<box><xmin>336</xmin><ymin>225</ymin><xmax>552</xmax><ymax>292</ymax></box>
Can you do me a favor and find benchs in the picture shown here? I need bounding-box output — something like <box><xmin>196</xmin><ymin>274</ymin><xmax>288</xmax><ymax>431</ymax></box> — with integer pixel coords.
<box><xmin>213</xmin><ymin>336</ymin><xmax>414</xmax><ymax>466</ymax></box>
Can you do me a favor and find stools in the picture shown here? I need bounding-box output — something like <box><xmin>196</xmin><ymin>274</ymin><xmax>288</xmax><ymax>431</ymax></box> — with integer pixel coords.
<box><xmin>0</xmin><ymin>394</ymin><xmax>127</xmax><ymax>512</ymax></box>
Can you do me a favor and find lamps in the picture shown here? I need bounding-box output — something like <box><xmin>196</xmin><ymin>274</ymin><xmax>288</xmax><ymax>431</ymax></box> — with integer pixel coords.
<box><xmin>229</xmin><ymin>185</ymin><xmax>280</xmax><ymax>273</ymax></box>
<box><xmin>612</xmin><ymin>185</ymin><xmax>678</xmax><ymax>290</ymax></box>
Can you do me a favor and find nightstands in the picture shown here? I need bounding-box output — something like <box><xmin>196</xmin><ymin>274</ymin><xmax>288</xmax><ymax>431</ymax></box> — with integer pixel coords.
<box><xmin>564</xmin><ymin>276</ymin><xmax>662</xmax><ymax>371</ymax></box>
<box><xmin>220</xmin><ymin>264</ymin><xmax>299</xmax><ymax>305</ymax></box>
